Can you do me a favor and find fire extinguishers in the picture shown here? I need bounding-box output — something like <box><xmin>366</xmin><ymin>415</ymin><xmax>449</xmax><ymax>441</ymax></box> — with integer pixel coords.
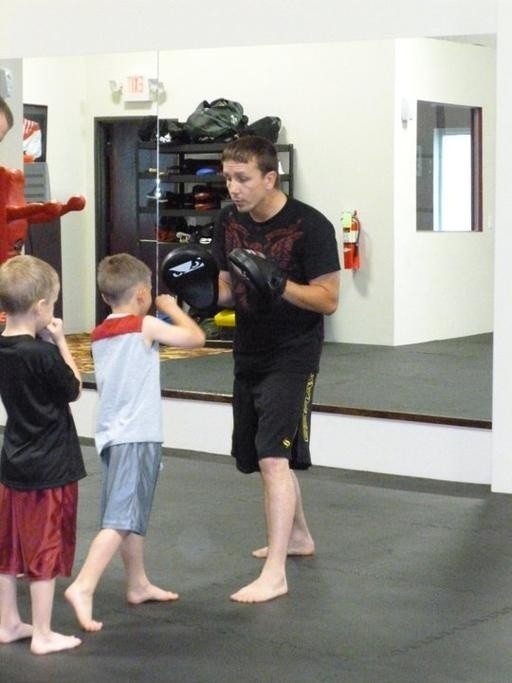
<box><xmin>342</xmin><ymin>211</ymin><xmax>360</xmax><ymax>269</ymax></box>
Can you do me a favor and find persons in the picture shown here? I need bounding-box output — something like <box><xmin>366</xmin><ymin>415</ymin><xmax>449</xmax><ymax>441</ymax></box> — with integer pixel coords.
<box><xmin>0</xmin><ymin>92</ymin><xmax>15</xmax><ymax>145</ymax></box>
<box><xmin>0</xmin><ymin>255</ymin><xmax>82</xmax><ymax>657</ymax></box>
<box><xmin>65</xmin><ymin>250</ymin><xmax>205</xmax><ymax>633</ymax></box>
<box><xmin>162</xmin><ymin>137</ymin><xmax>339</xmax><ymax>602</ymax></box>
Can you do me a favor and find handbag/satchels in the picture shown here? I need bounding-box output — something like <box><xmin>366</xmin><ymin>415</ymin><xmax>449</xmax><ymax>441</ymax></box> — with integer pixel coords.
<box><xmin>190</xmin><ymin>98</ymin><xmax>249</xmax><ymax>142</ymax></box>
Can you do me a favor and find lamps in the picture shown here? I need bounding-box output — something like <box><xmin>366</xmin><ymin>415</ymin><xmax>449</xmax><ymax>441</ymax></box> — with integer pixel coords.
<box><xmin>108</xmin><ymin>71</ymin><xmax>167</xmax><ymax>105</ymax></box>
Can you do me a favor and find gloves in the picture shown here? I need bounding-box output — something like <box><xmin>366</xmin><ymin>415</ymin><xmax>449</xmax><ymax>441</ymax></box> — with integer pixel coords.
<box><xmin>161</xmin><ymin>245</ymin><xmax>219</xmax><ymax>310</ymax></box>
<box><xmin>229</xmin><ymin>247</ymin><xmax>286</xmax><ymax>313</ymax></box>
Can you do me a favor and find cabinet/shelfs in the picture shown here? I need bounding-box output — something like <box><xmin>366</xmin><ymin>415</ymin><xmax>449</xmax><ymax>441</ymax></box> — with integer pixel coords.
<box><xmin>133</xmin><ymin>139</ymin><xmax>293</xmax><ymax>349</ymax></box>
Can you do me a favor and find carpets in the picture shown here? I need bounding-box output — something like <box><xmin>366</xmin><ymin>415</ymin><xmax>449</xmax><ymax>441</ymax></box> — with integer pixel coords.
<box><xmin>61</xmin><ymin>332</ymin><xmax>232</xmax><ymax>372</ymax></box>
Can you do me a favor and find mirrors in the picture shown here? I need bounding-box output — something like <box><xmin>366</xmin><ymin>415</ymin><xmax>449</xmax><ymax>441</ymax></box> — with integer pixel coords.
<box><xmin>415</xmin><ymin>97</ymin><xmax>484</xmax><ymax>232</ymax></box>
<box><xmin>1</xmin><ymin>33</ymin><xmax>496</xmax><ymax>426</ymax></box>
